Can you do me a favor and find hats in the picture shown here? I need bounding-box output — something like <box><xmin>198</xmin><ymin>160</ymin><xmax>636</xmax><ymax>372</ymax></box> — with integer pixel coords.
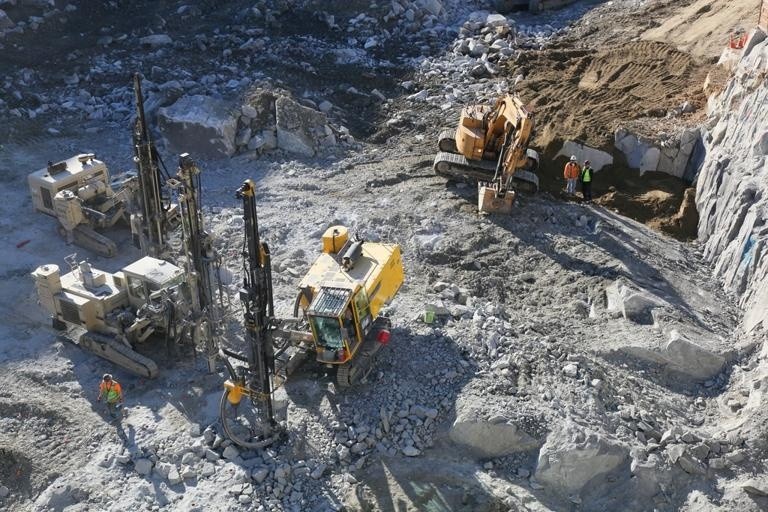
<box><xmin>102</xmin><ymin>374</ymin><xmax>113</xmax><ymax>382</ymax></box>
<box><xmin>583</xmin><ymin>160</ymin><xmax>591</xmax><ymax>166</ymax></box>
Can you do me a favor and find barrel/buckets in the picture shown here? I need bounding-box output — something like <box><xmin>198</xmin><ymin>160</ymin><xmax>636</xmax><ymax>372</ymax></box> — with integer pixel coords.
<box><xmin>423</xmin><ymin>311</ymin><xmax>436</xmax><ymax>324</ymax></box>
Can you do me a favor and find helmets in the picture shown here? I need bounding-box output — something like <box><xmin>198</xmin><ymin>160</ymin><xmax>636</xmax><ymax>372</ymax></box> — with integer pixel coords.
<box><xmin>570</xmin><ymin>155</ymin><xmax>577</xmax><ymax>161</ymax></box>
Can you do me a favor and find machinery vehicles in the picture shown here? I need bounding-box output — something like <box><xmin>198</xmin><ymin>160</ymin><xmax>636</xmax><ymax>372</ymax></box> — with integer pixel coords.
<box><xmin>433</xmin><ymin>93</ymin><xmax>543</xmax><ymax>218</ymax></box>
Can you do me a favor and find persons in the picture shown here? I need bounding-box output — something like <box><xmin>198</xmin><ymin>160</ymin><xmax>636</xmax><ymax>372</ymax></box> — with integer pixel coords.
<box><xmin>96</xmin><ymin>373</ymin><xmax>123</xmax><ymax>425</ymax></box>
<box><xmin>580</xmin><ymin>160</ymin><xmax>593</xmax><ymax>201</ymax></box>
<box><xmin>563</xmin><ymin>154</ymin><xmax>579</xmax><ymax>194</ymax></box>
<box><xmin>331</xmin><ymin>308</ymin><xmax>350</xmax><ymax>336</ymax></box>
